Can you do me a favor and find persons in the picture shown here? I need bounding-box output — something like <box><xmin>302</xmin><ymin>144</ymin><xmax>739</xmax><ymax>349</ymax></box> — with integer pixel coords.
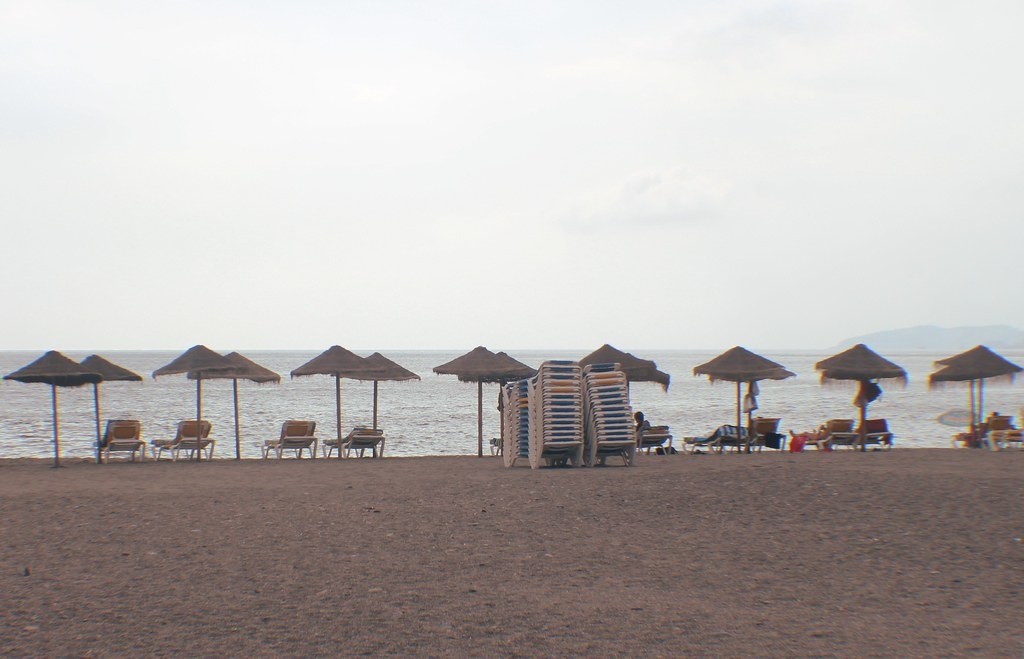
<box><xmin>634</xmin><ymin>412</ymin><xmax>650</xmax><ymax>432</ymax></box>
<box><xmin>790</xmin><ymin>425</ymin><xmax>824</xmax><ymax>440</ymax></box>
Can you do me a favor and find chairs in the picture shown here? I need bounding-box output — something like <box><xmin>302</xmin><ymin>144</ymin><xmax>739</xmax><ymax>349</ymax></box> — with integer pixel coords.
<box><xmin>503</xmin><ymin>360</ymin><xmax>637</xmax><ymax>470</ymax></box>
<box><xmin>950</xmin><ymin>416</ymin><xmax>1024</xmax><ymax>450</ymax></box>
<box><xmin>863</xmin><ymin>418</ymin><xmax>894</xmax><ymax>452</ymax></box>
<box><xmin>322</xmin><ymin>428</ymin><xmax>385</xmax><ymax>461</ymax></box>
<box><xmin>748</xmin><ymin>417</ymin><xmax>786</xmax><ymax>454</ymax></box>
<box><xmin>261</xmin><ymin>420</ymin><xmax>319</xmax><ymax>460</ymax></box>
<box><xmin>637</xmin><ymin>425</ymin><xmax>673</xmax><ymax>456</ymax></box>
<box><xmin>93</xmin><ymin>419</ymin><xmax>146</xmax><ymax>464</ymax></box>
<box><xmin>681</xmin><ymin>424</ymin><xmax>751</xmax><ymax>456</ymax></box>
<box><xmin>151</xmin><ymin>420</ymin><xmax>216</xmax><ymax>462</ymax></box>
<box><xmin>489</xmin><ymin>438</ymin><xmax>502</xmax><ymax>456</ymax></box>
<box><xmin>805</xmin><ymin>419</ymin><xmax>861</xmax><ymax>453</ymax></box>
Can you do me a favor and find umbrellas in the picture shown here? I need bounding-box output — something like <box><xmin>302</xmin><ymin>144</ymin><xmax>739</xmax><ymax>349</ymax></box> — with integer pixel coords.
<box><xmin>432</xmin><ymin>345</ymin><xmax>537</xmax><ymax>457</ymax></box>
<box><xmin>187</xmin><ymin>352</ymin><xmax>281</xmax><ymax>461</ymax></box>
<box><xmin>54</xmin><ymin>354</ymin><xmax>144</xmax><ymax>464</ymax></box>
<box><xmin>815</xmin><ymin>344</ymin><xmax>907</xmax><ymax>450</ymax></box>
<box><xmin>694</xmin><ymin>345</ymin><xmax>796</xmax><ymax>455</ymax></box>
<box><xmin>2</xmin><ymin>351</ymin><xmax>103</xmax><ymax>466</ymax></box>
<box><xmin>151</xmin><ymin>342</ymin><xmax>239</xmax><ymax>461</ymax></box>
<box><xmin>291</xmin><ymin>345</ymin><xmax>422</xmax><ymax>456</ymax></box>
<box><xmin>579</xmin><ymin>343</ymin><xmax>670</xmax><ymax>422</ymax></box>
<box><xmin>929</xmin><ymin>344</ymin><xmax>1023</xmax><ymax>450</ymax></box>
